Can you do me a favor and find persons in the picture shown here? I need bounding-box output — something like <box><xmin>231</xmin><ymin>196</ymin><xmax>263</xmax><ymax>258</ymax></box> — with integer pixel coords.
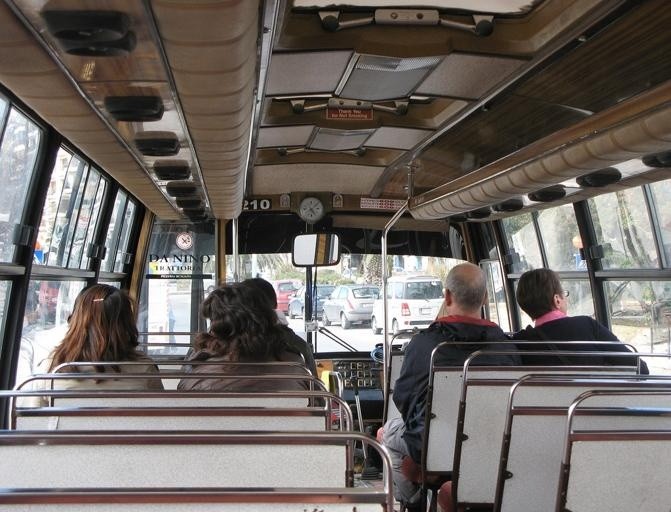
<box><xmin>381</xmin><ymin>262</ymin><xmax>521</xmax><ymax>512</ymax></box>
<box><xmin>512</xmin><ymin>268</ymin><xmax>649</xmax><ymax>381</ymax></box>
<box><xmin>177</xmin><ymin>278</ymin><xmax>326</xmax><ymax>393</ymax></box>
<box><xmin>30</xmin><ymin>285</ymin><xmax>164</xmax><ymax>407</ymax></box>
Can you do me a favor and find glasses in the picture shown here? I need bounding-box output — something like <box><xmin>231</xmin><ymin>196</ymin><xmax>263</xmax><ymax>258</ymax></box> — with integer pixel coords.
<box><xmin>560</xmin><ymin>289</ymin><xmax>570</xmax><ymax>297</ymax></box>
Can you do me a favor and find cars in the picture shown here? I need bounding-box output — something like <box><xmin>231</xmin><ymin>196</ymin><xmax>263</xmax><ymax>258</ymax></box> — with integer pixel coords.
<box><xmin>274</xmin><ymin>275</ymin><xmax>445</xmax><ymax>339</ymax></box>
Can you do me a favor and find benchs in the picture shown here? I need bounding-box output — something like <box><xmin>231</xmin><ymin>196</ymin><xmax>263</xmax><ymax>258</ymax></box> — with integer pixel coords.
<box><xmin>0</xmin><ymin>347</ymin><xmax>394</xmax><ymax>512</ymax></box>
<box><xmin>417</xmin><ymin>338</ymin><xmax>671</xmax><ymax>511</ymax></box>
